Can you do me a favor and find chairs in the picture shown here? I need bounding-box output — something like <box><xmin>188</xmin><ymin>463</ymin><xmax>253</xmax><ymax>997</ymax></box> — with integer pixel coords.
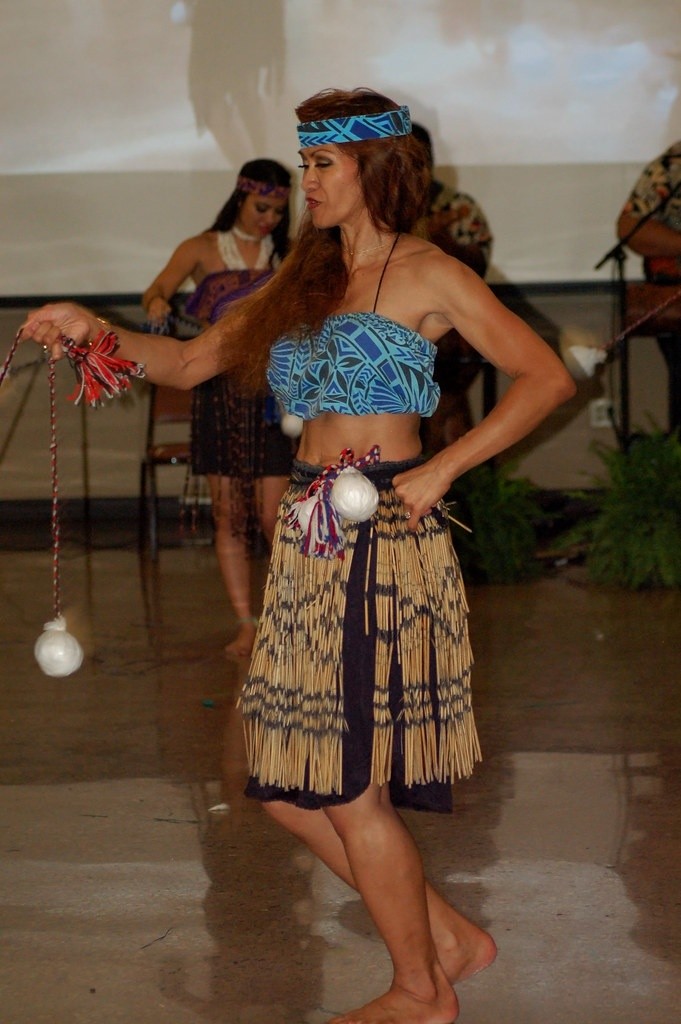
<box><xmin>137</xmin><ymin>336</ymin><xmax>198</xmax><ymax>561</ymax></box>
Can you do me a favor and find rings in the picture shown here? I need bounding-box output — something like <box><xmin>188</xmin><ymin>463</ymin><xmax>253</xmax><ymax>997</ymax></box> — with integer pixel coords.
<box><xmin>40</xmin><ymin>344</ymin><xmax>47</xmax><ymax>350</ymax></box>
<box><xmin>405</xmin><ymin>512</ymin><xmax>410</xmax><ymax>518</ymax></box>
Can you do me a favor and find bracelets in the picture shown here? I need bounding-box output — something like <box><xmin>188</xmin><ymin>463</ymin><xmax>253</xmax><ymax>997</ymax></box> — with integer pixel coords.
<box><xmin>97</xmin><ymin>316</ymin><xmax>112</xmax><ymax>333</ymax></box>
<box><xmin>145</xmin><ymin>293</ymin><xmax>163</xmax><ymax>313</ymax></box>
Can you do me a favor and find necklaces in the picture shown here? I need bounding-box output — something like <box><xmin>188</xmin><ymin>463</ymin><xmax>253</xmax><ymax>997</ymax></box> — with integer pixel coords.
<box><xmin>234</xmin><ymin>227</ymin><xmax>261</xmax><ymax>241</ymax></box>
<box><xmin>343</xmin><ymin>243</ymin><xmax>388</xmax><ymax>255</ymax></box>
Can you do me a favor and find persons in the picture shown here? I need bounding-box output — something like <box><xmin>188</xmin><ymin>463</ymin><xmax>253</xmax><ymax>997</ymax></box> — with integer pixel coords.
<box><xmin>402</xmin><ymin>121</ymin><xmax>491</xmax><ymax>431</ymax></box>
<box><xmin>616</xmin><ymin>140</ymin><xmax>681</xmax><ymax>435</ymax></box>
<box><xmin>142</xmin><ymin>159</ymin><xmax>296</xmax><ymax>655</ymax></box>
<box><xmin>19</xmin><ymin>86</ymin><xmax>576</xmax><ymax>1024</ymax></box>
